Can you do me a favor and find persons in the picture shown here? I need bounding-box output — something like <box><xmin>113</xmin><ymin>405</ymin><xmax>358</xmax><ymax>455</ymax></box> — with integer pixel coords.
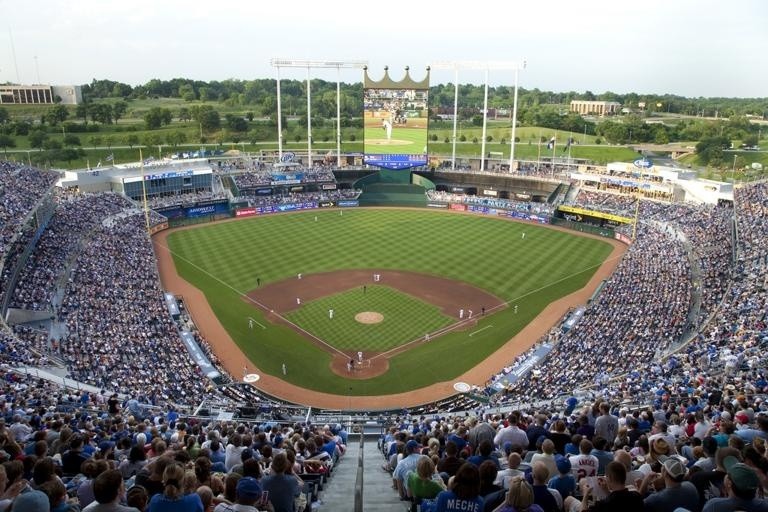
<box><xmin>0</xmin><ymin>157</ymin><xmax>233</xmax><ymax>510</ymax></box>
<box><xmin>328</xmin><ymin>307</ymin><xmax>334</xmax><ymax>319</ymax></box>
<box><xmin>243</xmin><ymin>365</ymin><xmax>248</xmax><ymax>377</ymax></box>
<box><xmin>296</xmin><ymin>295</ymin><xmax>300</xmax><ymax>304</ymax></box>
<box><xmin>232</xmin><ymin>377</ymin><xmax>768</xmax><ymax>512</ymax></box>
<box><xmin>247</xmin><ymin>319</ymin><xmax>253</xmax><ymax>329</ymax></box>
<box><xmin>493</xmin><ymin>179</ymin><xmax>767</xmax><ymax>376</ymax></box>
<box><xmin>297</xmin><ymin>272</ymin><xmax>302</xmax><ymax>280</ymax></box>
<box><xmin>227</xmin><ymin>149</ymin><xmax>568</xmax><ymax>220</ymax></box>
<box><xmin>347</xmin><ymin>358</ymin><xmax>355</xmax><ymax>372</ymax></box>
<box><xmin>256</xmin><ymin>277</ymin><xmax>260</xmax><ymax>286</ymax></box>
<box><xmin>467</xmin><ymin>308</ymin><xmax>473</xmax><ymax>318</ymax></box>
<box><xmin>481</xmin><ymin>307</ymin><xmax>485</xmax><ymax>316</ymax></box>
<box><xmin>423</xmin><ymin>332</ymin><xmax>431</xmax><ymax>342</ymax></box>
<box><xmin>459</xmin><ymin>307</ymin><xmax>464</xmax><ymax>319</ymax></box>
<box><xmin>280</xmin><ymin>363</ymin><xmax>287</xmax><ymax>376</ymax></box>
<box><xmin>361</xmin><ymin>87</ymin><xmax>427</xmax><ymax>142</ymax></box>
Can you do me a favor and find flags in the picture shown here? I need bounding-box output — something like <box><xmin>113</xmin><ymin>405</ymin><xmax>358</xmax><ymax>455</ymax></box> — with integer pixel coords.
<box><xmin>171</xmin><ymin>149</ymin><xmax>225</xmax><ymax>159</ymax></box>
<box><xmin>547</xmin><ymin>136</ymin><xmax>555</xmax><ymax>151</ymax></box>
<box><xmin>106</xmin><ymin>154</ymin><xmax>114</xmax><ymax>162</ymax></box>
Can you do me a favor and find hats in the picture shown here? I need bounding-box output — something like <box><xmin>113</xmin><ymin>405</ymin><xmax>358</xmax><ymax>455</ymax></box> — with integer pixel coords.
<box><xmin>555</xmin><ymin>443</ymin><xmax>577</xmax><ymax>470</ymax></box>
<box><xmin>653</xmin><ymin>438</ymin><xmax>686</xmax><ymax>478</ymax></box>
<box><xmin>236</xmin><ymin>476</ymin><xmax>261</xmax><ymax>499</ymax></box>
<box><xmin>406</xmin><ymin>440</ymin><xmax>422</xmax><ymax>447</ymax></box>
<box><xmin>275</xmin><ymin>436</ymin><xmax>283</xmax><ymax>444</ymax></box>
<box><xmin>721</xmin><ymin>411</ymin><xmax>748</xmax><ymax>422</ymax></box>
<box><xmin>98</xmin><ymin>441</ymin><xmax>115</xmax><ymax>448</ymax></box>
<box><xmin>723</xmin><ymin>456</ymin><xmax>757</xmax><ymax>491</ymax></box>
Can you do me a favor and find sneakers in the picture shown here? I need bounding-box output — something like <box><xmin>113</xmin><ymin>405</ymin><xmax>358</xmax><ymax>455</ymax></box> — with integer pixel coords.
<box><xmin>382</xmin><ymin>464</ymin><xmax>391</xmax><ymax>471</ymax></box>
